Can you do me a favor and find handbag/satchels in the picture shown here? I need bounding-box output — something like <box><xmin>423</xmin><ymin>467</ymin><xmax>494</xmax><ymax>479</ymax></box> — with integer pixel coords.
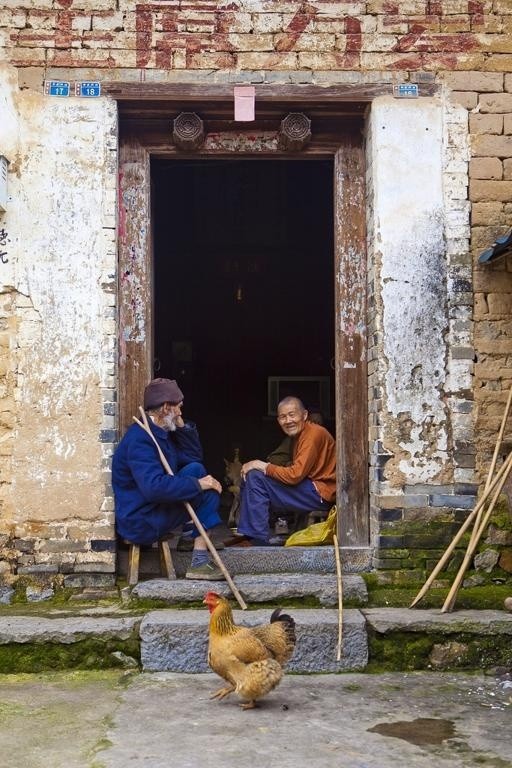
<box><xmin>284</xmin><ymin>504</ymin><xmax>337</xmax><ymax>547</ymax></box>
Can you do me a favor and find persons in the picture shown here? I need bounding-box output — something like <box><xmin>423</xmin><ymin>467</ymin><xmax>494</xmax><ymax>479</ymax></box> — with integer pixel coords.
<box><xmin>264</xmin><ymin>407</ymin><xmax>325</xmax><ymax>469</ymax></box>
<box><xmin>110</xmin><ymin>378</ymin><xmax>235</xmax><ymax>582</ymax></box>
<box><xmin>227</xmin><ymin>395</ymin><xmax>339</xmax><ymax>549</ymax></box>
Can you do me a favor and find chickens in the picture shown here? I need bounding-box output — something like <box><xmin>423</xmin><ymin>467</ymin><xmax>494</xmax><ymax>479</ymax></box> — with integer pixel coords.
<box><xmin>203</xmin><ymin>591</ymin><xmax>296</xmax><ymax>710</ymax></box>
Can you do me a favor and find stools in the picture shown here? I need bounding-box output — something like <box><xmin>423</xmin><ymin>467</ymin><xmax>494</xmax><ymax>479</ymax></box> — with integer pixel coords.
<box><xmin>124</xmin><ymin>530</ymin><xmax>178</xmax><ymax>587</ymax></box>
<box><xmin>298</xmin><ymin>510</ymin><xmax>331</xmax><ymax>531</ymax></box>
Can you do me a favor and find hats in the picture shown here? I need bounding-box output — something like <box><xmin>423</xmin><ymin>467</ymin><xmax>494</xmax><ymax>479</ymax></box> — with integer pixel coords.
<box><xmin>143</xmin><ymin>377</ymin><xmax>185</xmax><ymax>412</ymax></box>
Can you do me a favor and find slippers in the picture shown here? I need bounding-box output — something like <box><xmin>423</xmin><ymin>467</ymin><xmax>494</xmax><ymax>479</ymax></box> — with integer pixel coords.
<box><xmin>222</xmin><ymin>536</ymin><xmax>273</xmax><ymax>546</ymax></box>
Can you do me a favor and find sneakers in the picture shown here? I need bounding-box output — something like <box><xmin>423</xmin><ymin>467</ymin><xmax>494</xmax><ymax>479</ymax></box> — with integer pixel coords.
<box><xmin>176</xmin><ymin>537</ymin><xmax>226</xmax><ymax>552</ymax></box>
<box><xmin>184</xmin><ymin>560</ymin><xmax>234</xmax><ymax>581</ymax></box>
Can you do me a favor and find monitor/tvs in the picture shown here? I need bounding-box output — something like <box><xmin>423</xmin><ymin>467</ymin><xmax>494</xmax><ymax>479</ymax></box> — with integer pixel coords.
<box><xmin>267</xmin><ymin>375</ymin><xmax>331</xmax><ymax>419</ymax></box>
